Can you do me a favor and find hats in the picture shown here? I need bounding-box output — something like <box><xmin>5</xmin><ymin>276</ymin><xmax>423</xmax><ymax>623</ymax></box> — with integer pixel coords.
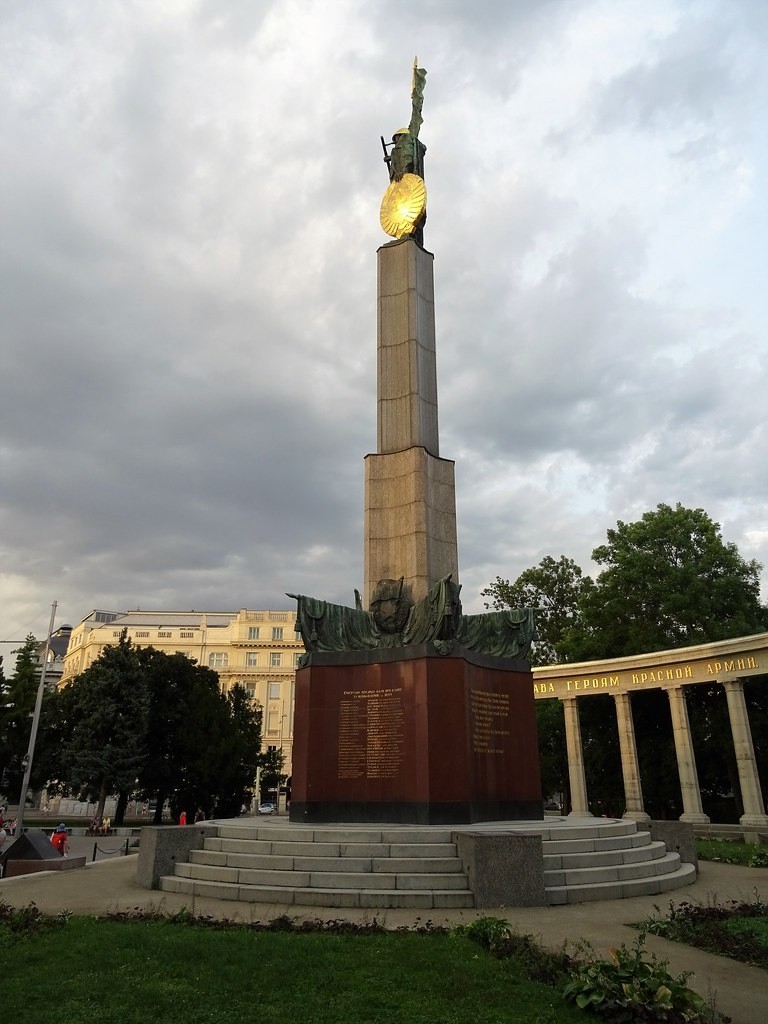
<box><xmin>57</xmin><ymin>823</ymin><xmax>66</xmax><ymax>831</ymax></box>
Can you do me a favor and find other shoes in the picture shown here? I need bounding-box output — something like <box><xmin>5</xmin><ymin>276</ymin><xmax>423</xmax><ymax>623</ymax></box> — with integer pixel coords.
<box><xmin>93</xmin><ymin>834</ymin><xmax>96</xmax><ymax>837</ymax></box>
<box><xmin>104</xmin><ymin>835</ymin><xmax>106</xmax><ymax>837</ymax></box>
<box><xmin>100</xmin><ymin>835</ymin><xmax>102</xmax><ymax>837</ymax></box>
<box><xmin>90</xmin><ymin>834</ymin><xmax>93</xmax><ymax>837</ymax></box>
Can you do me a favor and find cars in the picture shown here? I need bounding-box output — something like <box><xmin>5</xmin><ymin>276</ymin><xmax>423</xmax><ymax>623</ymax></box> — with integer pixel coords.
<box><xmin>258</xmin><ymin>803</ymin><xmax>277</xmax><ymax>815</ymax></box>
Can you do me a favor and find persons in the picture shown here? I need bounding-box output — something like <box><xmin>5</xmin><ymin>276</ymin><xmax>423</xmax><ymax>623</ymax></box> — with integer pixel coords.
<box><xmin>42</xmin><ymin>804</ymin><xmax>50</xmax><ymax>817</ymax></box>
<box><xmin>90</xmin><ymin>815</ymin><xmax>99</xmax><ymax>836</ymax></box>
<box><xmin>99</xmin><ymin>814</ymin><xmax>110</xmax><ymax>837</ymax></box>
<box><xmin>0</xmin><ymin>803</ymin><xmax>8</xmax><ymax>855</ymax></box>
<box><xmin>180</xmin><ymin>812</ymin><xmax>187</xmax><ymax>826</ymax></box>
<box><xmin>381</xmin><ymin>66</ymin><xmax>427</xmax><ymax>247</ymax></box>
<box><xmin>49</xmin><ymin>823</ymin><xmax>67</xmax><ymax>857</ymax></box>
<box><xmin>141</xmin><ymin>804</ymin><xmax>148</xmax><ymax>816</ymax></box>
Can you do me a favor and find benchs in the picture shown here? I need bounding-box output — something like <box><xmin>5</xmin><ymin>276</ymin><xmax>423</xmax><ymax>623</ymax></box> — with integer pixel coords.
<box><xmin>4</xmin><ymin>826</ymin><xmax>142</xmax><ymax>838</ymax></box>
